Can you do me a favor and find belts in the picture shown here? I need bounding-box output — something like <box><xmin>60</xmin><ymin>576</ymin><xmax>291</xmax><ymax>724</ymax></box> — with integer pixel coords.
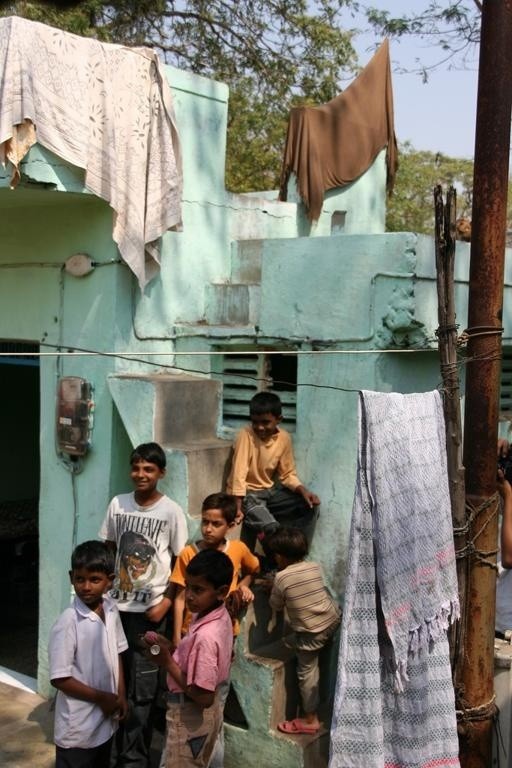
<box><xmin>164</xmin><ymin>692</ymin><xmax>196</xmax><ymax>703</ymax></box>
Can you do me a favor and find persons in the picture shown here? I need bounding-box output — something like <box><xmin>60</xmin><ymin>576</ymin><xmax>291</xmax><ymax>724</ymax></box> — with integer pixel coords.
<box><xmin>136</xmin><ymin>549</ymin><xmax>233</xmax><ymax>768</ymax></box>
<box><xmin>494</xmin><ymin>465</ymin><xmax>512</xmax><ymax>643</ymax></box>
<box><xmin>266</xmin><ymin>524</ymin><xmax>342</xmax><ymax>735</ymax></box>
<box><xmin>224</xmin><ymin>392</ymin><xmax>320</xmax><ymax>572</ymax></box>
<box><xmin>166</xmin><ymin>492</ymin><xmax>262</xmax><ymax>659</ymax></box>
<box><xmin>46</xmin><ymin>539</ymin><xmax>130</xmax><ymax>768</ymax></box>
<box><xmin>98</xmin><ymin>441</ymin><xmax>188</xmax><ymax>701</ymax></box>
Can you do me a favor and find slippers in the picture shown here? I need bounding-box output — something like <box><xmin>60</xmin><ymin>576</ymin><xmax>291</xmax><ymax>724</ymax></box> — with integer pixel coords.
<box><xmin>278</xmin><ymin>717</ymin><xmax>319</xmax><ymax>732</ymax></box>
